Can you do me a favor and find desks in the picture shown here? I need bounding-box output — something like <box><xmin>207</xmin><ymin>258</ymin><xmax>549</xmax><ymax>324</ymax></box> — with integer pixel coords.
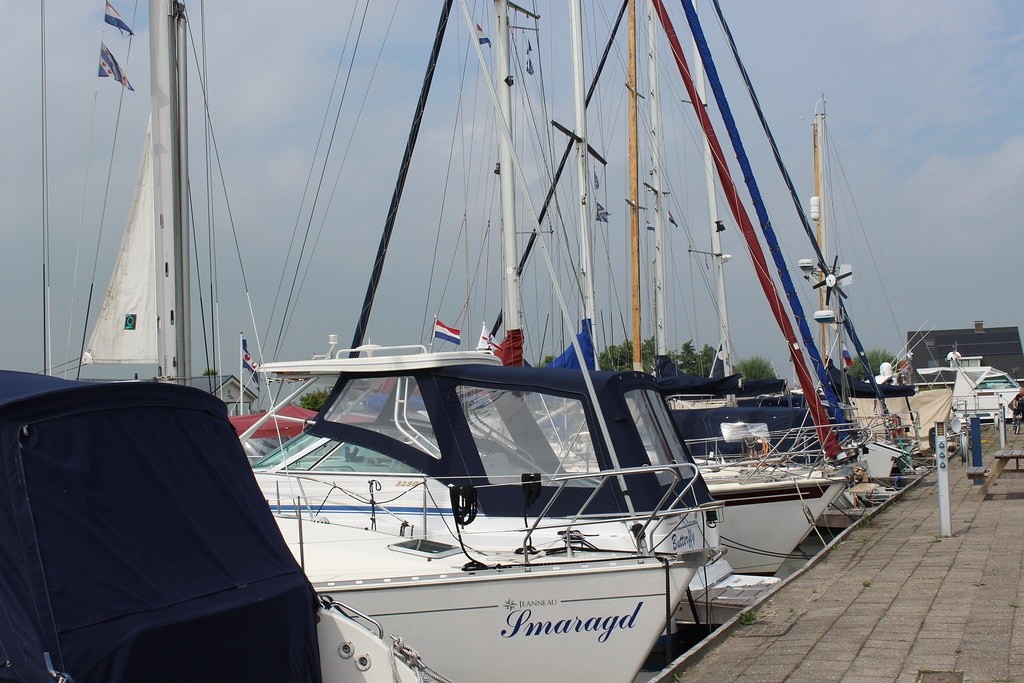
<box><xmin>847</xmin><ymin>483</ymin><xmax>880</xmax><ymax>509</ymax></box>
<box><xmin>985</xmin><ymin>449</ymin><xmax>1024</xmax><ymax>492</ymax></box>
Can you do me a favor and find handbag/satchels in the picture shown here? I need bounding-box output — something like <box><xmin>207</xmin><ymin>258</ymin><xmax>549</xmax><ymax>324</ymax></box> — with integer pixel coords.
<box><xmin>1008</xmin><ymin>400</ymin><xmax>1014</xmax><ymax>411</ymax></box>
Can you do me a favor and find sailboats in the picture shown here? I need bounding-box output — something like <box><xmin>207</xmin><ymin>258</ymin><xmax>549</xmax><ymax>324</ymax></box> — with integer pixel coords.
<box><xmin>84</xmin><ymin>0</ymin><xmax>954</xmax><ymax>683</ymax></box>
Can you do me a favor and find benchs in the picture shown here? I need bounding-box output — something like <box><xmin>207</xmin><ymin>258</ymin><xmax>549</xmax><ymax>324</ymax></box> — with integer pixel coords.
<box><xmin>966</xmin><ymin>467</ymin><xmax>987</xmax><ymax>485</ymax></box>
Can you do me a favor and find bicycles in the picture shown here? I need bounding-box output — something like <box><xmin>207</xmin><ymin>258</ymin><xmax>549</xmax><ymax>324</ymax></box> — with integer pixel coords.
<box><xmin>1010</xmin><ymin>408</ymin><xmax>1023</xmax><ymax>434</ymax></box>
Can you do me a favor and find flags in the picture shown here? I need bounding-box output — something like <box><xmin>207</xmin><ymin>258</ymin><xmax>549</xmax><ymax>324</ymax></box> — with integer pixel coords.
<box><xmin>435</xmin><ymin>319</ymin><xmax>460</xmax><ymax>344</ymax></box>
<box><xmin>527</xmin><ymin>58</ymin><xmax>534</xmax><ymax>75</ymax></box>
<box><xmin>99</xmin><ymin>43</ymin><xmax>134</xmax><ymax>91</ymax></box>
<box><xmin>597</xmin><ymin>203</ymin><xmax>612</xmax><ymax>223</ymax></box>
<box><xmin>595</xmin><ymin>175</ymin><xmax>599</xmax><ymax>191</ymax></box>
<box><xmin>479</xmin><ymin>326</ymin><xmax>502</xmax><ymax>351</ymax></box>
<box><xmin>476</xmin><ymin>23</ymin><xmax>492</xmax><ymax>47</ymax></box>
<box><xmin>668</xmin><ymin>211</ymin><xmax>678</xmax><ymax>227</ymax></box>
<box><xmin>841</xmin><ymin>341</ymin><xmax>854</xmax><ymax>368</ymax></box>
<box><xmin>105</xmin><ymin>0</ymin><xmax>134</xmax><ymax>36</ymax></box>
<box><xmin>646</xmin><ymin>220</ymin><xmax>654</xmax><ymax>231</ymax></box>
<box><xmin>243</xmin><ymin>339</ymin><xmax>260</xmax><ymax>391</ymax></box>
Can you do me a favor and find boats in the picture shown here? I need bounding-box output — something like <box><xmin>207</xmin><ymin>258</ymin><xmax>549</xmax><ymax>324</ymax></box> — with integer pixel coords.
<box><xmin>0</xmin><ymin>367</ymin><xmax>456</xmax><ymax>683</ymax></box>
<box><xmin>916</xmin><ymin>366</ymin><xmax>1022</xmax><ymax>419</ymax></box>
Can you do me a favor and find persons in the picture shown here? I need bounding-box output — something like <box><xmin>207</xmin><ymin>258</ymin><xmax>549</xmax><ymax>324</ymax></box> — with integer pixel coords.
<box><xmin>947</xmin><ymin>346</ymin><xmax>961</xmax><ymax>368</ymax></box>
<box><xmin>1011</xmin><ymin>388</ymin><xmax>1024</xmax><ymax>427</ymax></box>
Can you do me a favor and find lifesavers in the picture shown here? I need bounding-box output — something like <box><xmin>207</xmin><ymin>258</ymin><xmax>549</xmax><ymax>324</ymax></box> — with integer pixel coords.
<box><xmin>891</xmin><ymin>413</ymin><xmax>902</xmax><ymax>439</ymax></box>
